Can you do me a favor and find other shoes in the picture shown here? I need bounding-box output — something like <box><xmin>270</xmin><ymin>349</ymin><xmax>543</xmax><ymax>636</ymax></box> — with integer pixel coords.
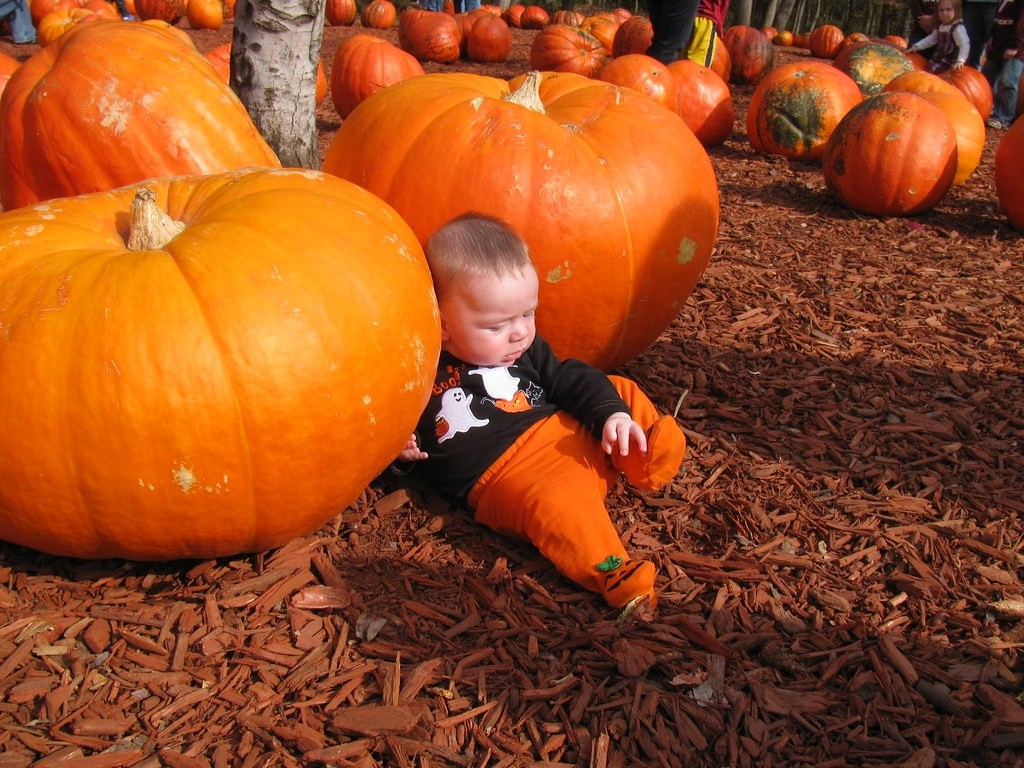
<box><xmin>987</xmin><ymin>118</ymin><xmax>1012</xmax><ymax>129</ymax></box>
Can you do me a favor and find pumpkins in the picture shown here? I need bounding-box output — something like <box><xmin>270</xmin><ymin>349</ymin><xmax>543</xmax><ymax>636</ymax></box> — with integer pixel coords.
<box><xmin>0</xmin><ymin>0</ymin><xmax>1024</xmax><ymax>560</ymax></box>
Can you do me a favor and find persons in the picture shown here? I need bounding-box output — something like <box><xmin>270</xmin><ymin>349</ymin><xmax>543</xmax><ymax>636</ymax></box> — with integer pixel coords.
<box><xmin>644</xmin><ymin>1</ymin><xmax>731</xmax><ymax>68</ymax></box>
<box><xmin>387</xmin><ymin>210</ymin><xmax>687</xmax><ymax>610</ymax></box>
<box><xmin>0</xmin><ymin>0</ymin><xmax>36</xmax><ymax>44</ymax></box>
<box><xmin>106</xmin><ymin>0</ymin><xmax>135</xmax><ymax>22</ymax></box>
<box><xmin>418</xmin><ymin>0</ymin><xmax>481</xmax><ymax>13</ymax></box>
<box><xmin>905</xmin><ymin>0</ymin><xmax>1023</xmax><ymax>132</ymax></box>
